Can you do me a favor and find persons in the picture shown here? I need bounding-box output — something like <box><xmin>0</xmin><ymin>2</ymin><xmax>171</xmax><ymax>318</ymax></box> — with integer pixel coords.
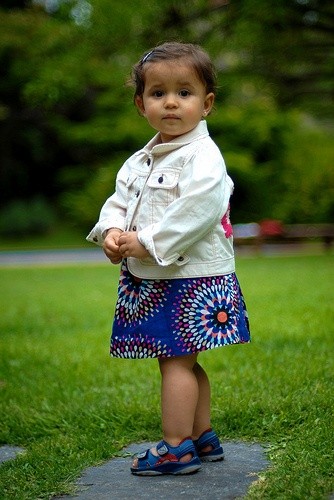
<box><xmin>88</xmin><ymin>40</ymin><xmax>250</xmax><ymax>481</ymax></box>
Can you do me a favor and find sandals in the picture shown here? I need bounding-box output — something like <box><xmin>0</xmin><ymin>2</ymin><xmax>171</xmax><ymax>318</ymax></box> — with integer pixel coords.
<box><xmin>130</xmin><ymin>437</ymin><xmax>201</xmax><ymax>477</ymax></box>
<box><xmin>194</xmin><ymin>428</ymin><xmax>225</xmax><ymax>462</ymax></box>
